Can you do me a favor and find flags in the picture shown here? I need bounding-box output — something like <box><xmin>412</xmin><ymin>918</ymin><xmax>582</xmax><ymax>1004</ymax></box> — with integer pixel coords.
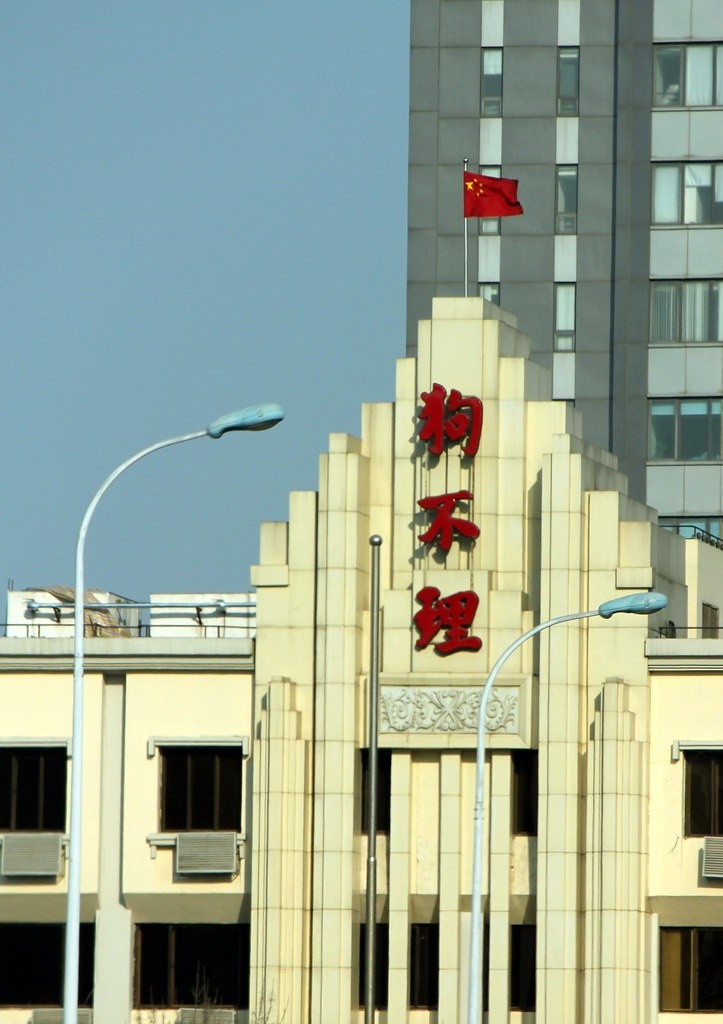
<box><xmin>462</xmin><ymin>168</ymin><xmax>523</xmax><ymax>218</ymax></box>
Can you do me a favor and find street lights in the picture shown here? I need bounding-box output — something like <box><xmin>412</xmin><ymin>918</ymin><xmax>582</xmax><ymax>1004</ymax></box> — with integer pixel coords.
<box><xmin>62</xmin><ymin>403</ymin><xmax>283</xmax><ymax>1024</ymax></box>
<box><xmin>464</xmin><ymin>591</ymin><xmax>669</xmax><ymax>1024</ymax></box>
